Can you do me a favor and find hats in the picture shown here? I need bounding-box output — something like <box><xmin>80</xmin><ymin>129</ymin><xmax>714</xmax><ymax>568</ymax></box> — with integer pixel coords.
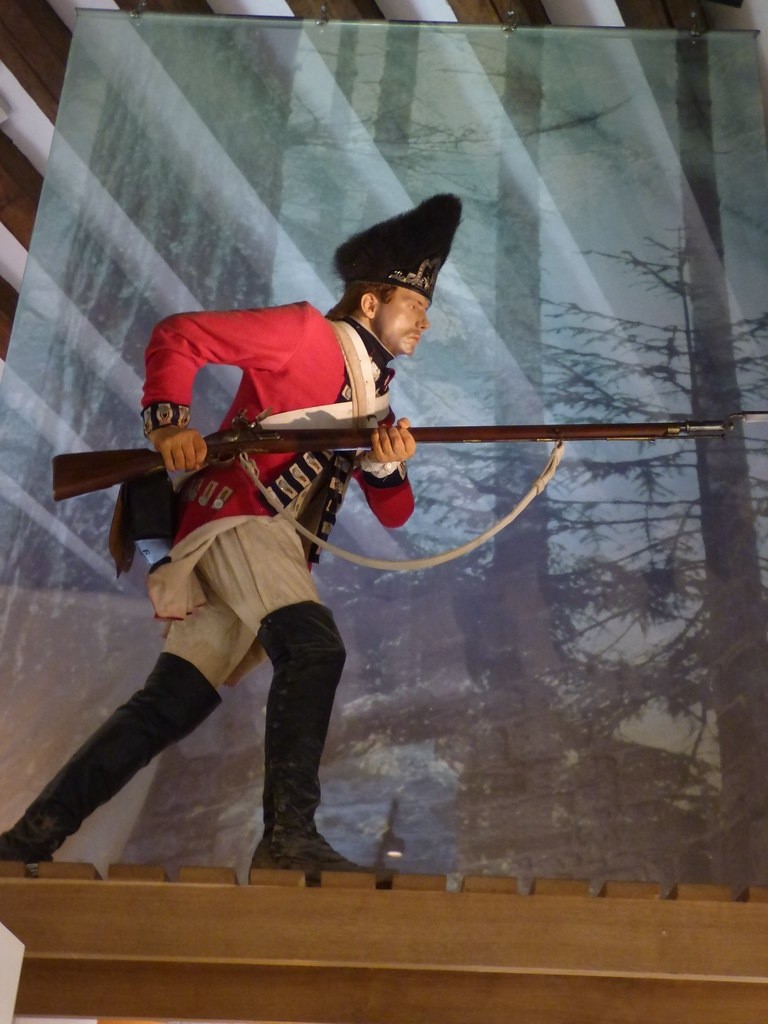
<box><xmin>334</xmin><ymin>192</ymin><xmax>462</xmax><ymax>304</ymax></box>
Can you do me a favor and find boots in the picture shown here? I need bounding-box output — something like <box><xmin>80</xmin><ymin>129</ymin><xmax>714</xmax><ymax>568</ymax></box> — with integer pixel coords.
<box><xmin>0</xmin><ymin>651</ymin><xmax>223</xmax><ymax>876</ymax></box>
<box><xmin>248</xmin><ymin>601</ymin><xmax>399</xmax><ymax>888</ymax></box>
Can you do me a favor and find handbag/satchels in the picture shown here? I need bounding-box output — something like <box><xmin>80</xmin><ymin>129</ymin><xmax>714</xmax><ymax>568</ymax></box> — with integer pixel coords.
<box><xmin>107</xmin><ymin>476</ymin><xmax>175</xmax><ymax>579</ymax></box>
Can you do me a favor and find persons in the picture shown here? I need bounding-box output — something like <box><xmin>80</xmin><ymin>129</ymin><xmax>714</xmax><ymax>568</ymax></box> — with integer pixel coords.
<box><xmin>0</xmin><ymin>194</ymin><xmax>462</xmax><ymax>868</ymax></box>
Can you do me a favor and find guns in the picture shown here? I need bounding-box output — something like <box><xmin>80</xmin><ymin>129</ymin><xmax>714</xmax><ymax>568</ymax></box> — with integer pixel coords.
<box><xmin>52</xmin><ymin>406</ymin><xmax>768</xmax><ymax>503</ymax></box>
